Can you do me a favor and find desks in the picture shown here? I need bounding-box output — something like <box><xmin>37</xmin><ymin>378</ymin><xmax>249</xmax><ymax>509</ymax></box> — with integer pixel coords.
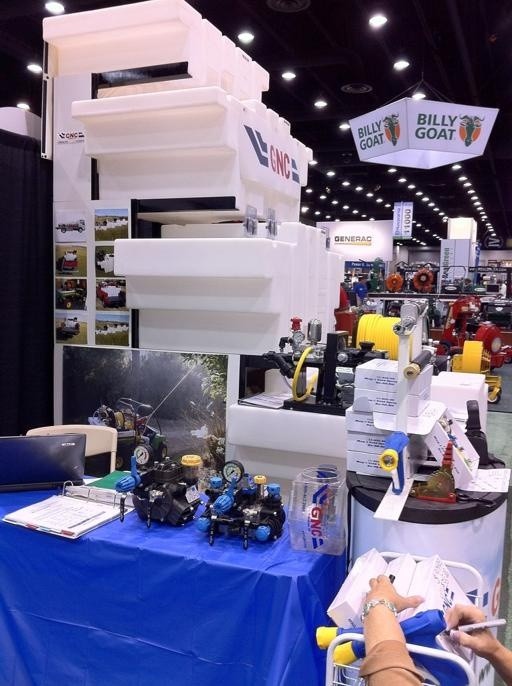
<box><xmin>1</xmin><ymin>475</ymin><xmax>348</xmax><ymax>686</ymax></box>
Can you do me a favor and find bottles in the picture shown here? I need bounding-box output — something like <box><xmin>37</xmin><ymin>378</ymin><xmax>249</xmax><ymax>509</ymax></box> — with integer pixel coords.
<box><xmin>182</xmin><ymin>454</ymin><xmax>203</xmax><ymax>485</ymax></box>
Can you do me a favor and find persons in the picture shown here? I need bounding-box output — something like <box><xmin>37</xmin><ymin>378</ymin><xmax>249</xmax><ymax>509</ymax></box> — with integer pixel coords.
<box><xmin>357</xmin><ymin>573</ymin><xmax>512</xmax><ymax>686</ymax></box>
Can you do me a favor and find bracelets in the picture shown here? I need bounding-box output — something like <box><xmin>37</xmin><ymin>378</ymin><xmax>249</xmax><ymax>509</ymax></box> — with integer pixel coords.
<box><xmin>360</xmin><ymin>599</ymin><xmax>398</xmax><ymax>622</ymax></box>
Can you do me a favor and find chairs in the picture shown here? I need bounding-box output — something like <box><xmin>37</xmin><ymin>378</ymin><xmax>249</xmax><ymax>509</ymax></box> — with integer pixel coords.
<box><xmin>24</xmin><ymin>424</ymin><xmax>117</xmax><ymax>474</ymax></box>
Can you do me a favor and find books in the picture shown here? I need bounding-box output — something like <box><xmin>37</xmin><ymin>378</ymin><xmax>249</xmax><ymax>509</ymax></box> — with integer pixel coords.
<box><xmin>2</xmin><ymin>469</ymin><xmax>136</xmax><ymax>541</ymax></box>
